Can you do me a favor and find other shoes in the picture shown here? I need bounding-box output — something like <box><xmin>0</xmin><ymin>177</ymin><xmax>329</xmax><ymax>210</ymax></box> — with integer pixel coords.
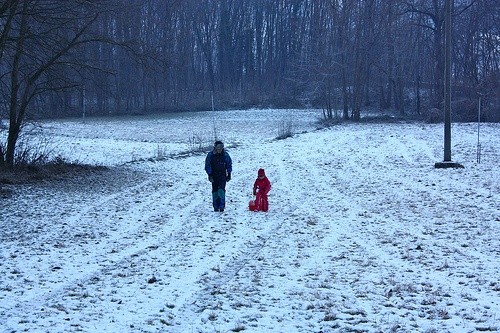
<box><xmin>220</xmin><ymin>209</ymin><xmax>223</xmax><ymax>213</ymax></box>
<box><xmin>264</xmin><ymin>208</ymin><xmax>267</xmax><ymax>212</ymax></box>
<box><xmin>254</xmin><ymin>209</ymin><xmax>260</xmax><ymax>212</ymax></box>
<box><xmin>214</xmin><ymin>209</ymin><xmax>218</xmax><ymax>212</ymax></box>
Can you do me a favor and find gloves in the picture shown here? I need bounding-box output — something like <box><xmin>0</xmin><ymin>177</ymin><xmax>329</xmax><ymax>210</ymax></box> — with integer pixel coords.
<box><xmin>209</xmin><ymin>174</ymin><xmax>215</xmax><ymax>182</ymax></box>
<box><xmin>253</xmin><ymin>191</ymin><xmax>257</xmax><ymax>195</ymax></box>
<box><xmin>225</xmin><ymin>174</ymin><xmax>230</xmax><ymax>182</ymax></box>
<box><xmin>262</xmin><ymin>189</ymin><xmax>266</xmax><ymax>196</ymax></box>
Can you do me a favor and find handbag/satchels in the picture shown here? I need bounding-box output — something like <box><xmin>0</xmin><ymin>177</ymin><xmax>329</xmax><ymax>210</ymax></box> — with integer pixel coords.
<box><xmin>250</xmin><ymin>200</ymin><xmax>256</xmax><ymax>210</ymax></box>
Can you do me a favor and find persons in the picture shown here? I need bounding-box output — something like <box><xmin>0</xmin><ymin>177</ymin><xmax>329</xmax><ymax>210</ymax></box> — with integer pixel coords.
<box><xmin>252</xmin><ymin>168</ymin><xmax>272</xmax><ymax>213</ymax></box>
<box><xmin>205</xmin><ymin>140</ymin><xmax>233</xmax><ymax>213</ymax></box>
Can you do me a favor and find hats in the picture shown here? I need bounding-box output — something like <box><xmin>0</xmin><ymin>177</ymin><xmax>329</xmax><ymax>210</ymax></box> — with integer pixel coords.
<box><xmin>258</xmin><ymin>169</ymin><xmax>265</xmax><ymax>175</ymax></box>
<box><xmin>214</xmin><ymin>140</ymin><xmax>222</xmax><ymax>148</ymax></box>
